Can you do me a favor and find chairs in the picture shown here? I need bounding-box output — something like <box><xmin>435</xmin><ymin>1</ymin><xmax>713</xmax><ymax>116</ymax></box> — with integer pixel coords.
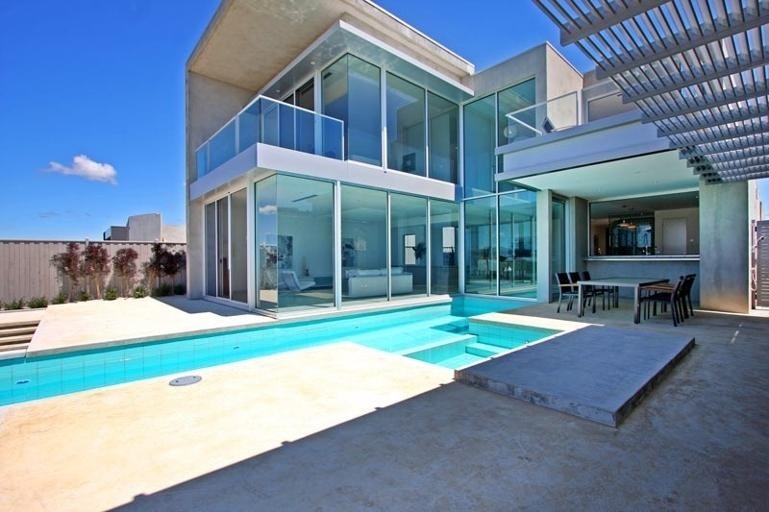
<box><xmin>556</xmin><ymin>272</ymin><xmax>696</xmax><ymax>327</ymax></box>
<box><xmin>281</xmin><ymin>270</ymin><xmax>315</xmax><ymax>292</ymax></box>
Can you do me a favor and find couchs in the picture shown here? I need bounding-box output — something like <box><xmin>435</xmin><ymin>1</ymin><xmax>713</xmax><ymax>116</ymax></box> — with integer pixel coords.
<box><xmin>346</xmin><ymin>267</ymin><xmax>414</xmax><ymax>296</ymax></box>
